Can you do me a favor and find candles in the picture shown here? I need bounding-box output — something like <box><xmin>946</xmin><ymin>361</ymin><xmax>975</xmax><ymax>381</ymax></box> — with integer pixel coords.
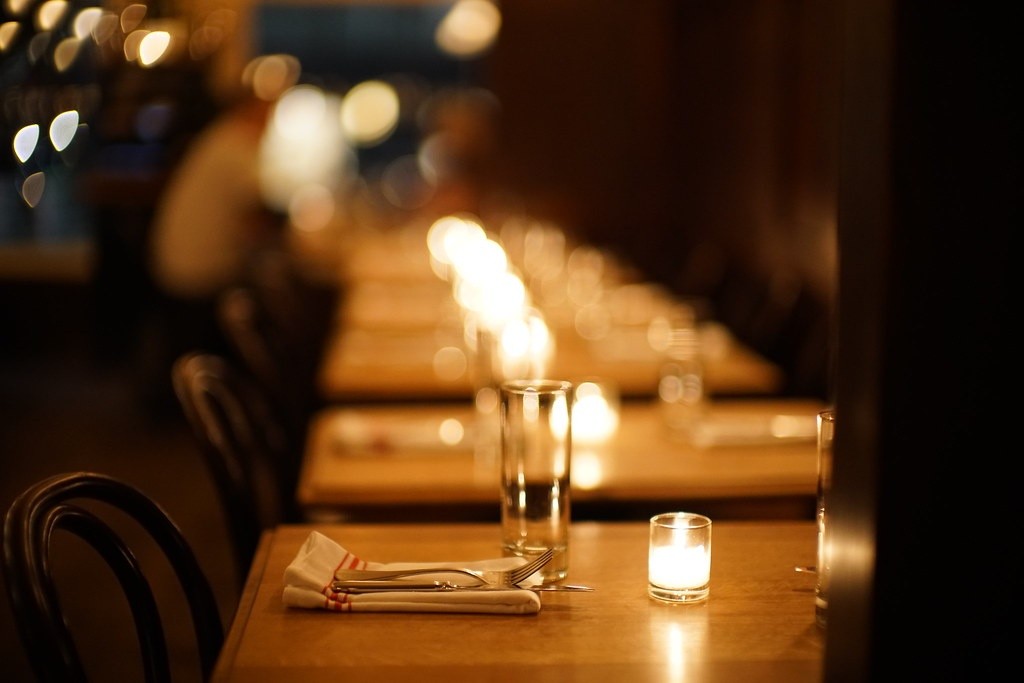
<box><xmin>649</xmin><ymin>514</ymin><xmax>711</xmax><ymax>589</ymax></box>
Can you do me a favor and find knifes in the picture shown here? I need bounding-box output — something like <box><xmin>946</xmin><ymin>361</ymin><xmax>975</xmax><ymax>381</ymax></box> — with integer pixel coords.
<box><xmin>335</xmin><ymin>579</ymin><xmax>595</xmax><ymax>594</ymax></box>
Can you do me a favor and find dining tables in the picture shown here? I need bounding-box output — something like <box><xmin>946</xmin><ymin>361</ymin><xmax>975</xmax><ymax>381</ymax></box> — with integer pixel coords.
<box><xmin>211</xmin><ymin>520</ymin><xmax>823</xmax><ymax>683</ymax></box>
<box><xmin>300</xmin><ymin>223</ymin><xmax>831</xmax><ymax>506</ymax></box>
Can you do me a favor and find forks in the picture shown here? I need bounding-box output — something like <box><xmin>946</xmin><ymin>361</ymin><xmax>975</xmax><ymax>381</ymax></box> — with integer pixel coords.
<box><xmin>332</xmin><ymin>548</ymin><xmax>554</xmax><ymax>584</ymax></box>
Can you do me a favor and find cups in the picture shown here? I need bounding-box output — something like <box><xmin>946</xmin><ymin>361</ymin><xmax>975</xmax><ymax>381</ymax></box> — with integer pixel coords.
<box><xmin>499</xmin><ymin>377</ymin><xmax>572</xmax><ymax>581</ymax></box>
<box><xmin>645</xmin><ymin>511</ymin><xmax>713</xmax><ymax>607</ymax></box>
<box><xmin>814</xmin><ymin>409</ymin><xmax>835</xmax><ymax>628</ymax></box>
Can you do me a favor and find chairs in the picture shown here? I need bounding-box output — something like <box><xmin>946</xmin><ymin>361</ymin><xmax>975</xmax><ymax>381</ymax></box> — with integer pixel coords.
<box><xmin>172</xmin><ymin>352</ymin><xmax>296</xmax><ymax>600</ymax></box>
<box><xmin>2</xmin><ymin>472</ymin><xmax>226</xmax><ymax>683</ymax></box>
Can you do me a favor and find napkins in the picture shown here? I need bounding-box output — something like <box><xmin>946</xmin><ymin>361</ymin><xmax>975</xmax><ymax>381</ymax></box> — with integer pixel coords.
<box><xmin>281</xmin><ymin>531</ymin><xmax>545</xmax><ymax>614</ymax></box>
